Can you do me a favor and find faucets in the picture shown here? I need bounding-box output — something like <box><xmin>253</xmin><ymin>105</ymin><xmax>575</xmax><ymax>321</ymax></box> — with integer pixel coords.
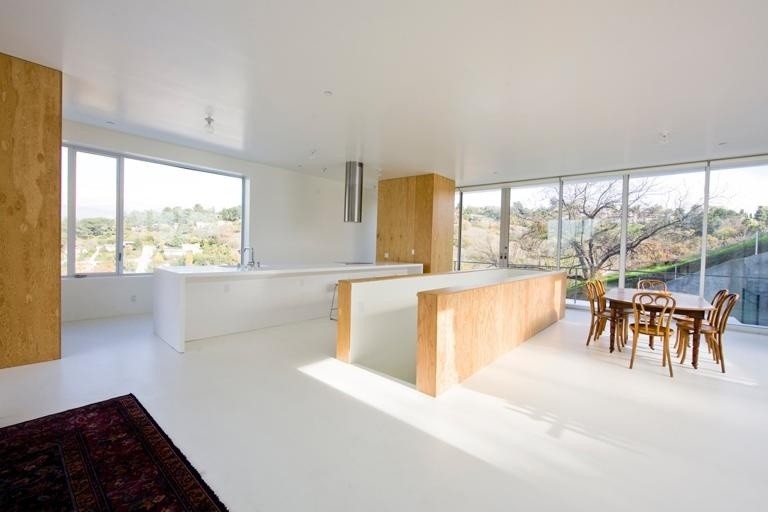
<box><xmin>243</xmin><ymin>247</ymin><xmax>254</xmax><ymax>266</ymax></box>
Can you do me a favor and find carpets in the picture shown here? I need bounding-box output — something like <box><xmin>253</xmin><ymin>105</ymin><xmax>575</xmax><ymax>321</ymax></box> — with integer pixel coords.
<box><xmin>0</xmin><ymin>391</ymin><xmax>230</xmax><ymax>512</ymax></box>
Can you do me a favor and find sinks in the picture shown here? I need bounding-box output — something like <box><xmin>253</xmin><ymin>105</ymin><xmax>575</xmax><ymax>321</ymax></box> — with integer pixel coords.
<box><xmin>219</xmin><ymin>266</ymin><xmax>246</xmax><ymax>269</ymax></box>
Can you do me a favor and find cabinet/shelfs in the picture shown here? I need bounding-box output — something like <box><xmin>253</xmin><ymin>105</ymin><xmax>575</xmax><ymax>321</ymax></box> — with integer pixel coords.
<box><xmin>375</xmin><ymin>174</ymin><xmax>457</xmax><ymax>275</ymax></box>
<box><xmin>155</xmin><ymin>263</ymin><xmax>424</xmax><ymax>355</ymax></box>
<box><xmin>0</xmin><ymin>52</ymin><xmax>62</xmax><ymax>370</ymax></box>
<box><xmin>338</xmin><ymin>271</ymin><xmax>504</xmax><ymax>363</ymax></box>
<box><xmin>417</xmin><ymin>271</ymin><xmax>567</xmax><ymax>397</ymax></box>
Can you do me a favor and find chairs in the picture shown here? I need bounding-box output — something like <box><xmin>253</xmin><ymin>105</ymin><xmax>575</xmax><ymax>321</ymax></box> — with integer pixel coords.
<box><xmin>585</xmin><ymin>278</ymin><xmax>740</xmax><ymax>378</ymax></box>
